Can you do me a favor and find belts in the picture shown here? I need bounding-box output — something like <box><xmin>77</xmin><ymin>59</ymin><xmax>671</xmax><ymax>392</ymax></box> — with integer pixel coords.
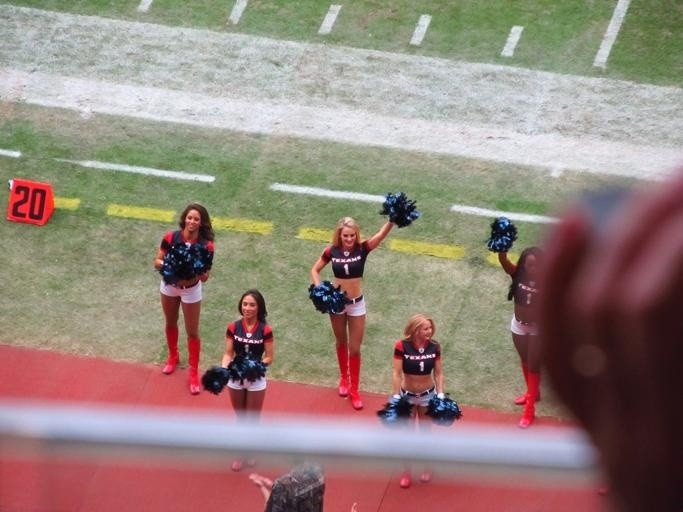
<box><xmin>347</xmin><ymin>294</ymin><xmax>363</xmax><ymax>305</ymax></box>
<box><xmin>170</xmin><ymin>279</ymin><xmax>201</xmax><ymax>290</ymax></box>
<box><xmin>516</xmin><ymin>317</ymin><xmax>538</xmax><ymax>324</ymax></box>
<box><xmin>401</xmin><ymin>386</ymin><xmax>434</xmax><ymax>398</ymax></box>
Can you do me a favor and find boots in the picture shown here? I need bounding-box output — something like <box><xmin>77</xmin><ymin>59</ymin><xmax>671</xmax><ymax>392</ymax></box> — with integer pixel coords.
<box><xmin>515</xmin><ymin>361</ymin><xmax>541</xmax><ymax>428</ymax></box>
<box><xmin>336</xmin><ymin>342</ymin><xmax>350</xmax><ymax>396</ymax></box>
<box><xmin>186</xmin><ymin>337</ymin><xmax>201</xmax><ymax>394</ymax></box>
<box><xmin>163</xmin><ymin>326</ymin><xmax>179</xmax><ymax>374</ymax></box>
<box><xmin>347</xmin><ymin>354</ymin><xmax>365</xmax><ymax>410</ymax></box>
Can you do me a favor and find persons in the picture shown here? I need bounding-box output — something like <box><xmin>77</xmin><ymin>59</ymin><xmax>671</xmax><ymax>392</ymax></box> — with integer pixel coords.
<box><xmin>537</xmin><ymin>170</ymin><xmax>683</xmax><ymax>512</ymax></box>
<box><xmin>220</xmin><ymin>290</ymin><xmax>274</xmax><ymax>472</ymax></box>
<box><xmin>311</xmin><ymin>216</ymin><xmax>394</xmax><ymax>410</ymax></box>
<box><xmin>497</xmin><ymin>241</ymin><xmax>546</xmax><ymax>430</ymax></box>
<box><xmin>152</xmin><ymin>205</ymin><xmax>214</xmax><ymax>395</ymax></box>
<box><xmin>250</xmin><ymin>456</ymin><xmax>325</xmax><ymax>512</ymax></box>
<box><xmin>391</xmin><ymin>314</ymin><xmax>443</xmax><ymax>489</ymax></box>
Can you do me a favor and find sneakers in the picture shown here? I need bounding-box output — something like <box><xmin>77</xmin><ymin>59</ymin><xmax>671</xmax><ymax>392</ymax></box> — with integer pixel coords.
<box><xmin>230</xmin><ymin>454</ymin><xmax>257</xmax><ymax>471</ymax></box>
<box><xmin>419</xmin><ymin>467</ymin><xmax>432</xmax><ymax>483</ymax></box>
<box><xmin>399</xmin><ymin>467</ymin><xmax>412</xmax><ymax>488</ymax></box>
